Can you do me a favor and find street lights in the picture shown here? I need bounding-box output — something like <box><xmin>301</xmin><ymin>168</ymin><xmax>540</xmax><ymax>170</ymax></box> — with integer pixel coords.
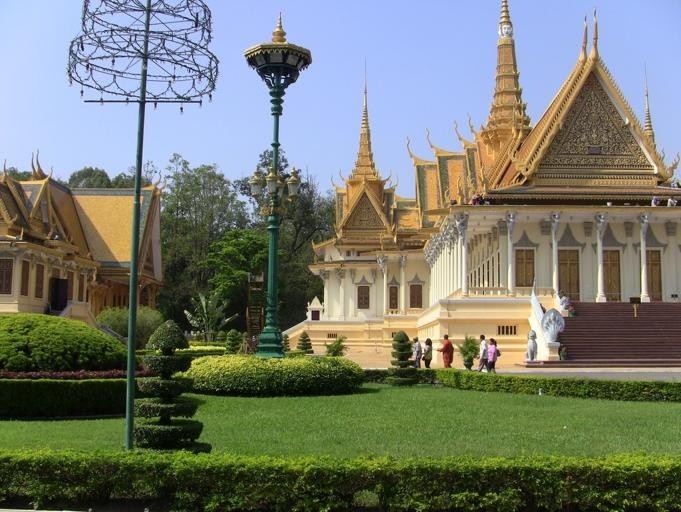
<box><xmin>249</xmin><ymin>165</ymin><xmax>302</xmax><ymax>358</ymax></box>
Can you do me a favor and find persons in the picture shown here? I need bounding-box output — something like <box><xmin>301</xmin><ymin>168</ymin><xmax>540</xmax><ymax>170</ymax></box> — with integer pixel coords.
<box><xmin>422</xmin><ymin>338</ymin><xmax>433</xmax><ymax>368</ymax></box>
<box><xmin>488</xmin><ymin>337</ymin><xmax>498</xmax><ymax>373</ymax></box>
<box><xmin>478</xmin><ymin>335</ymin><xmax>489</xmax><ymax>372</ymax></box>
<box><xmin>436</xmin><ymin>334</ymin><xmax>455</xmax><ymax>368</ymax></box>
<box><xmin>413</xmin><ymin>336</ymin><xmax>423</xmax><ymax>368</ymax></box>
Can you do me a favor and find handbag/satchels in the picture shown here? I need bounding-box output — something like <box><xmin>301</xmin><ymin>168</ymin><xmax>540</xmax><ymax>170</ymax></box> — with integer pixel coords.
<box><xmin>496</xmin><ymin>349</ymin><xmax>500</xmax><ymax>356</ymax></box>
